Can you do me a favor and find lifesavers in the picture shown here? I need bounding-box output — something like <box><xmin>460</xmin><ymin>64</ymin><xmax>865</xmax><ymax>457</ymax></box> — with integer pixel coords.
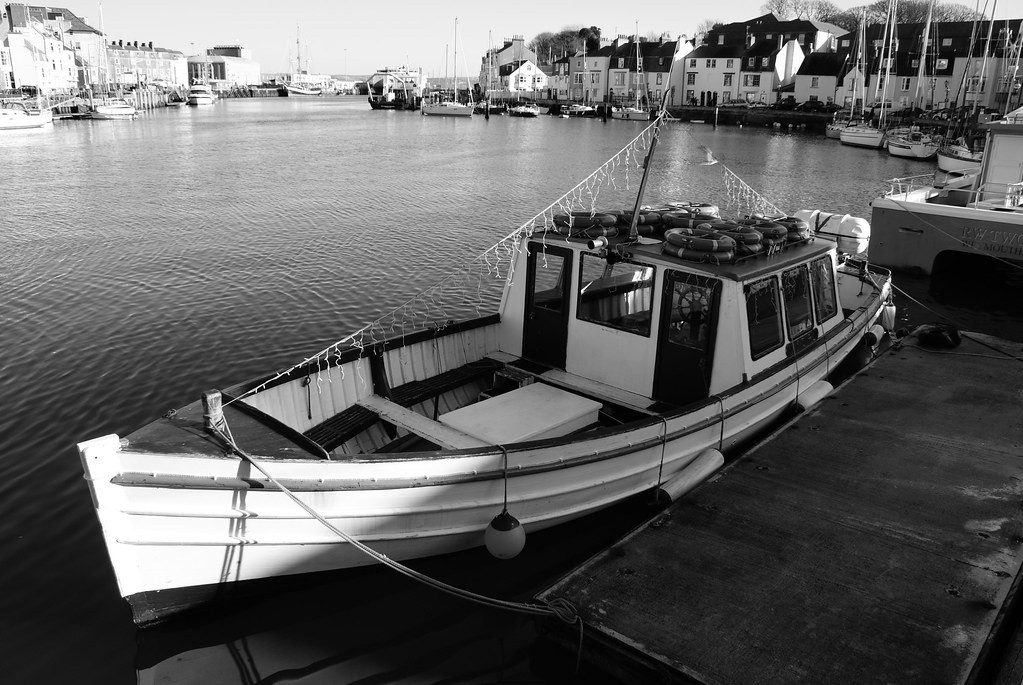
<box><xmin>552</xmin><ymin>199</ymin><xmax>813</xmax><ymax>263</ymax></box>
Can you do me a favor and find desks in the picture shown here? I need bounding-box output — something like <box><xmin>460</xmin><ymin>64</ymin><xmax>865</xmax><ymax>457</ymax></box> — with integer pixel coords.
<box><xmin>437</xmin><ymin>382</ymin><xmax>602</xmax><ymax>445</ymax></box>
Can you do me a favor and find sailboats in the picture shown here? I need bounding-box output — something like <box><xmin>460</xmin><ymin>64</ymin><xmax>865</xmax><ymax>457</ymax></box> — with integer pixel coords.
<box><xmin>473</xmin><ymin>30</ymin><xmax>505</xmax><ymax>114</ymax></box>
<box><xmin>826</xmin><ymin>11</ymin><xmax>866</xmax><ymax>139</ymax></box>
<box><xmin>840</xmin><ymin>0</ymin><xmax>899</xmax><ymax>149</ymax></box>
<box><xmin>287</xmin><ymin>26</ymin><xmax>321</xmax><ymax>96</ymax></box>
<box><xmin>90</xmin><ymin>1</ymin><xmax>135</xmax><ymax>119</ymax></box>
<box><xmin>507</xmin><ymin>47</ymin><xmax>540</xmax><ymax>117</ymax></box>
<box><xmin>611</xmin><ymin>22</ymin><xmax>651</xmax><ymax>121</ymax></box>
<box><xmin>559</xmin><ymin>40</ymin><xmax>597</xmax><ymax>118</ymax></box>
<box><xmin>937</xmin><ymin>0</ymin><xmax>996</xmax><ymax>177</ymax></box>
<box><xmin>423</xmin><ymin>18</ymin><xmax>477</xmax><ymax>116</ymax></box>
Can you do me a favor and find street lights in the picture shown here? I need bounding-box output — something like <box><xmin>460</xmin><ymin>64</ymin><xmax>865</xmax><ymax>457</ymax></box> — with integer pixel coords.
<box><xmin>344</xmin><ymin>49</ymin><xmax>347</xmax><ymax>81</ymax></box>
<box><xmin>931</xmin><ymin>79</ymin><xmax>937</xmax><ymax>108</ymax></box>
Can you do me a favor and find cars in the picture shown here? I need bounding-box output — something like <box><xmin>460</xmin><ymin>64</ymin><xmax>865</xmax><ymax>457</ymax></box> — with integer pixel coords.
<box><xmin>716</xmin><ymin>99</ymin><xmax>748</xmax><ymax>109</ymax></box>
<box><xmin>889</xmin><ymin>107</ymin><xmax>926</xmax><ymax>118</ymax></box>
<box><xmin>793</xmin><ymin>100</ymin><xmax>823</xmax><ymax>112</ymax></box>
<box><xmin>864</xmin><ymin>102</ymin><xmax>892</xmax><ymax>116</ymax></box>
<box><xmin>767</xmin><ymin>98</ymin><xmax>796</xmax><ymax>110</ymax></box>
<box><xmin>8</xmin><ymin>85</ymin><xmax>42</xmax><ymax>95</ymax></box>
<box><xmin>919</xmin><ymin>106</ymin><xmax>985</xmax><ymax>123</ymax></box>
<box><xmin>746</xmin><ymin>101</ymin><xmax>769</xmax><ymax>110</ymax></box>
<box><xmin>812</xmin><ymin>104</ymin><xmax>842</xmax><ymax>113</ymax></box>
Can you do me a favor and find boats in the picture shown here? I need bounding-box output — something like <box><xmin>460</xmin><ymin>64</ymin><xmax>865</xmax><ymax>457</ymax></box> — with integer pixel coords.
<box><xmin>367</xmin><ymin>61</ymin><xmax>423</xmax><ymax>110</ymax></box>
<box><xmin>0</xmin><ymin>101</ymin><xmax>53</xmax><ymax>130</ymax></box>
<box><xmin>188</xmin><ymin>80</ymin><xmax>213</xmax><ymax>105</ymax></box>
<box><xmin>887</xmin><ymin>126</ymin><xmax>939</xmax><ymax>158</ymax></box>
<box><xmin>77</xmin><ymin>89</ymin><xmax>891</xmax><ymax>629</ymax></box>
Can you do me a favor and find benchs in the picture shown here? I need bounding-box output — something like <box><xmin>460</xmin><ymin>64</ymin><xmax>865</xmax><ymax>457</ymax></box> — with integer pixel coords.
<box><xmin>483</xmin><ymin>350</ymin><xmax>671</xmax><ymax>417</ymax></box>
<box><xmin>356</xmin><ymin>394</ymin><xmax>496</xmax><ymax>450</ymax></box>
